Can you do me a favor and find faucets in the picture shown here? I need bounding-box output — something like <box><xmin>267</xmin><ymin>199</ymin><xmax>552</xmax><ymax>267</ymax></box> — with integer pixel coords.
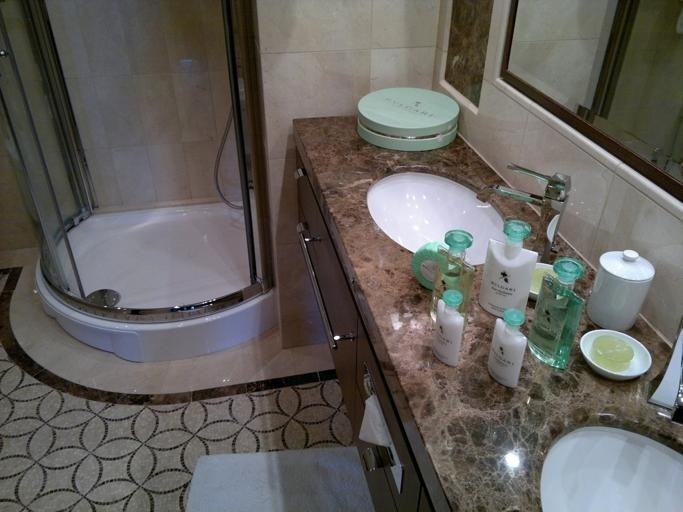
<box><xmin>648</xmin><ymin>314</ymin><xmax>683</xmax><ymax>411</ymax></box>
<box><xmin>494</xmin><ymin>161</ymin><xmax>572</xmax><ymax>263</ymax></box>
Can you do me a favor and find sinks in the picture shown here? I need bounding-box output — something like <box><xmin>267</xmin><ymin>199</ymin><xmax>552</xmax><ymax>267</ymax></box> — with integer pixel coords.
<box><xmin>541</xmin><ymin>425</ymin><xmax>682</xmax><ymax>511</ymax></box>
<box><xmin>367</xmin><ymin>173</ymin><xmax>505</xmax><ymax>265</ymax></box>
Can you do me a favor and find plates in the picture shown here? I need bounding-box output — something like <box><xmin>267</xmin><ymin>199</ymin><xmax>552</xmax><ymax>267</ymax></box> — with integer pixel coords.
<box><xmin>580</xmin><ymin>330</ymin><xmax>652</xmax><ymax>379</ymax></box>
<box><xmin>528</xmin><ymin>261</ymin><xmax>553</xmax><ymax>302</ymax></box>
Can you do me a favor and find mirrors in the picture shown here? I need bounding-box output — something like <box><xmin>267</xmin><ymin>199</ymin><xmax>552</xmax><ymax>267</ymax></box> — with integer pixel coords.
<box><xmin>500</xmin><ymin>0</ymin><xmax>683</xmax><ymax>203</ymax></box>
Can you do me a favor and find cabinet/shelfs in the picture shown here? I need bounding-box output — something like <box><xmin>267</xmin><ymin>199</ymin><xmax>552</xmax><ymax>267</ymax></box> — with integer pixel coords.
<box><xmin>297</xmin><ymin>149</ymin><xmax>435</xmax><ymax>512</ymax></box>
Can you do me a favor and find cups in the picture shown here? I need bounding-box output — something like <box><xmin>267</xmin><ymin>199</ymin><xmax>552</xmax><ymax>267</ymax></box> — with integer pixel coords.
<box><xmin>586</xmin><ymin>248</ymin><xmax>655</xmax><ymax>331</ymax></box>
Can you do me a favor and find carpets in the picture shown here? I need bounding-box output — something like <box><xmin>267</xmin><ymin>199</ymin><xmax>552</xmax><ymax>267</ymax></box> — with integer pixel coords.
<box><xmin>185</xmin><ymin>445</ymin><xmax>375</xmax><ymax>512</ymax></box>
